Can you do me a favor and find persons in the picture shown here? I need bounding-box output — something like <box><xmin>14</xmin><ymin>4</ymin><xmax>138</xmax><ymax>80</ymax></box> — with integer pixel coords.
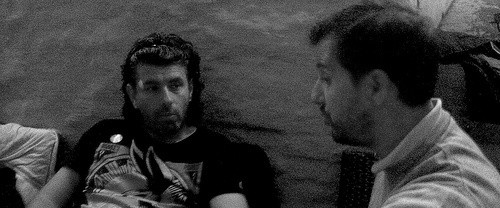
<box><xmin>27</xmin><ymin>32</ymin><xmax>248</xmax><ymax>208</ymax></box>
<box><xmin>307</xmin><ymin>1</ymin><xmax>500</xmax><ymax>208</ymax></box>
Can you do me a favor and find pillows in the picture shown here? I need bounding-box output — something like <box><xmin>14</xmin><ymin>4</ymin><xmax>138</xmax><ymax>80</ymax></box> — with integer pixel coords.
<box><xmin>0</xmin><ymin>121</ymin><xmax>60</xmax><ymax>208</ymax></box>
